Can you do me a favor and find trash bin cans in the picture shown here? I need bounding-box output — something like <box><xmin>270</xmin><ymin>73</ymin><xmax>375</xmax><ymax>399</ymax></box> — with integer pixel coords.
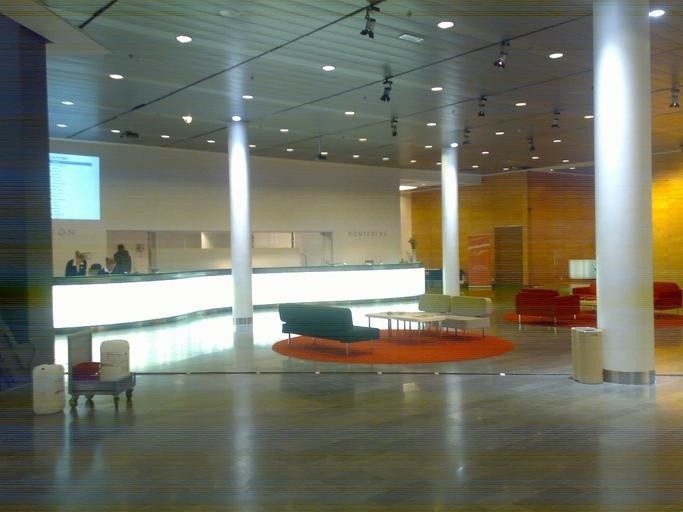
<box><xmin>570</xmin><ymin>326</ymin><xmax>603</xmax><ymax>385</ymax></box>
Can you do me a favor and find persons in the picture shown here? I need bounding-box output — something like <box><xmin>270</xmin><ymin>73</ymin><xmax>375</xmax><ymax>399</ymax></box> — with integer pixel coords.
<box><xmin>98</xmin><ymin>257</ymin><xmax>118</xmax><ymax>274</ymax></box>
<box><xmin>65</xmin><ymin>251</ymin><xmax>87</xmax><ymax>277</ymax></box>
<box><xmin>113</xmin><ymin>244</ymin><xmax>131</xmax><ymax>274</ymax></box>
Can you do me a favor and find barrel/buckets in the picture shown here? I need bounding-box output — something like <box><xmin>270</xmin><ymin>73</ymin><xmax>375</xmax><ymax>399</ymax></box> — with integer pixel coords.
<box><xmin>30</xmin><ymin>363</ymin><xmax>66</xmax><ymax>416</ymax></box>
<box><xmin>101</xmin><ymin>339</ymin><xmax>130</xmax><ymax>376</ymax></box>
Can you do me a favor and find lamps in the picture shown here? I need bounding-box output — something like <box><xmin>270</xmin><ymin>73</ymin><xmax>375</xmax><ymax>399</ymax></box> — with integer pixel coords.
<box><xmin>391</xmin><ymin>115</ymin><xmax>398</xmax><ymax>136</ymax></box>
<box><xmin>494</xmin><ymin>40</ymin><xmax>510</xmax><ymax>69</ymax></box>
<box><xmin>359</xmin><ymin>2</ymin><xmax>382</xmax><ymax>40</ymax></box>
<box><xmin>551</xmin><ymin>109</ymin><xmax>560</xmax><ymax>128</ymax></box>
<box><xmin>669</xmin><ymin>84</ymin><xmax>681</xmax><ymax>109</ymax></box>
<box><xmin>379</xmin><ymin>75</ymin><xmax>393</xmax><ymax>102</ymax></box>
<box><xmin>478</xmin><ymin>94</ymin><xmax>487</xmax><ymax>117</ymax></box>
<box><xmin>464</xmin><ymin>128</ymin><xmax>470</xmax><ymax>144</ymax></box>
<box><xmin>526</xmin><ymin>136</ymin><xmax>535</xmax><ymax>151</ymax></box>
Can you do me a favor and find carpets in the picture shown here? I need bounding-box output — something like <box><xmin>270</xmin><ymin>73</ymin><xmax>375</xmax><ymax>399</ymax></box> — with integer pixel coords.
<box><xmin>272</xmin><ymin>329</ymin><xmax>512</xmax><ymax>364</ymax></box>
<box><xmin>502</xmin><ymin>310</ymin><xmax>683</xmax><ymax>330</ymax></box>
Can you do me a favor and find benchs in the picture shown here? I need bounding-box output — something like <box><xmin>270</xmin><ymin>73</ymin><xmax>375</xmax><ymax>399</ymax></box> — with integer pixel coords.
<box><xmin>419</xmin><ymin>294</ymin><xmax>491</xmax><ymax>337</ymax></box>
<box><xmin>572</xmin><ymin>281</ymin><xmax>596</xmax><ymax>301</ymax></box>
<box><xmin>279</xmin><ymin>303</ymin><xmax>380</xmax><ymax>357</ymax></box>
<box><xmin>515</xmin><ymin>288</ymin><xmax>580</xmax><ymax>323</ymax></box>
<box><xmin>653</xmin><ymin>280</ymin><xmax>683</xmax><ymax>315</ymax></box>
